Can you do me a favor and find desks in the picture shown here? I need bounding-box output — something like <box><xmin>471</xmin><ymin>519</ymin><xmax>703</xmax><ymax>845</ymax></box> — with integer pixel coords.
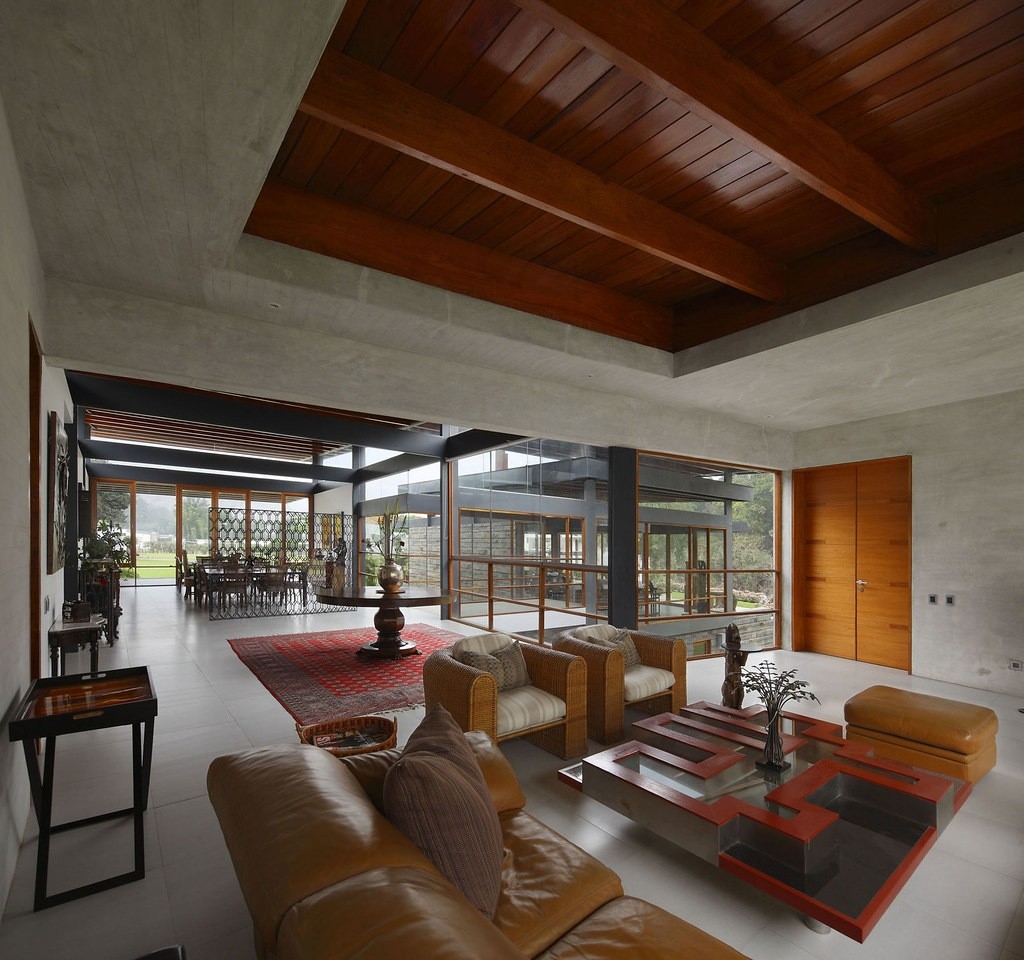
<box><xmin>49</xmin><ymin>612</ymin><xmax>105</xmax><ymax>679</ymax></box>
<box><xmin>80</xmin><ymin>558</ymin><xmax>123</xmax><ymax>648</ymax></box>
<box><xmin>314</xmin><ymin>585</ymin><xmax>459</xmax><ymax>660</ymax></box>
<box><xmin>557</xmin><ymin>699</ymin><xmax>974</xmax><ymax>943</ymax></box>
<box><xmin>191</xmin><ymin>562</ymin><xmax>307</xmax><ymax>612</ymax></box>
<box><xmin>8</xmin><ymin>664</ymin><xmax>157</xmax><ymax>912</ymax></box>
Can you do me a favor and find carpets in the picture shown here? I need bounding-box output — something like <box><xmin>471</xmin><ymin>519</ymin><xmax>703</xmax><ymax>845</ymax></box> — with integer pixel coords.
<box><xmin>226</xmin><ymin>622</ymin><xmax>467</xmax><ymax>726</ymax></box>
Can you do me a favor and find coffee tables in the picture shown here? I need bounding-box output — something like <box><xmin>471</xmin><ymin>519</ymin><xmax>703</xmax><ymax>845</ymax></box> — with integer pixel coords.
<box><xmin>720</xmin><ymin>623</ymin><xmax>764</xmax><ymax>709</ymax></box>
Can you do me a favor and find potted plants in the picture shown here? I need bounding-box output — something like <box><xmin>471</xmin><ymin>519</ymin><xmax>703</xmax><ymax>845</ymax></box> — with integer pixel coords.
<box><xmin>77</xmin><ymin>516</ymin><xmax>133</xmax><ymax>582</ymax></box>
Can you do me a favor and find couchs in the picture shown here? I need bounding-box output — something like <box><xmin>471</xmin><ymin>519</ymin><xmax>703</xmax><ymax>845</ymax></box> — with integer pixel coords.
<box><xmin>206</xmin><ymin>729</ymin><xmax>752</xmax><ymax>959</ymax></box>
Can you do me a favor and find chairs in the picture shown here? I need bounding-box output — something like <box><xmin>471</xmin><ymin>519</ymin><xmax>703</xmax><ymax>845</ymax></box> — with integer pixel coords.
<box><xmin>175</xmin><ymin>549</ymin><xmax>303</xmax><ymax>613</ymax></box>
<box><xmin>423</xmin><ymin>633</ymin><xmax>587</xmax><ymax>760</ymax></box>
<box><xmin>552</xmin><ymin>625</ymin><xmax>687</xmax><ymax>744</ymax></box>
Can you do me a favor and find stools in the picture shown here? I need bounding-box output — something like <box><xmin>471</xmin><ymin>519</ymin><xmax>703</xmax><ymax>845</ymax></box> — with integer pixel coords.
<box><xmin>844</xmin><ymin>686</ymin><xmax>999</xmax><ymax>784</ymax></box>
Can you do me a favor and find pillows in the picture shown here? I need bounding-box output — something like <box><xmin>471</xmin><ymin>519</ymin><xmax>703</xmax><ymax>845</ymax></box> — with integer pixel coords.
<box><xmin>460</xmin><ymin>641</ymin><xmax>532</xmax><ymax>695</ymax></box>
<box><xmin>589</xmin><ymin>627</ymin><xmax>643</xmax><ymax>668</ymax></box>
<box><xmin>381</xmin><ymin>704</ymin><xmax>507</xmax><ymax>923</ymax></box>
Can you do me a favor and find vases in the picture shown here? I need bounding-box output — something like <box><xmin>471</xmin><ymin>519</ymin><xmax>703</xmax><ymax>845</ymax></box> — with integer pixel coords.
<box><xmin>377</xmin><ymin>559</ymin><xmax>404</xmax><ymax>592</ymax></box>
<box><xmin>765</xmin><ymin>702</ymin><xmax>784</xmax><ymax>765</ymax></box>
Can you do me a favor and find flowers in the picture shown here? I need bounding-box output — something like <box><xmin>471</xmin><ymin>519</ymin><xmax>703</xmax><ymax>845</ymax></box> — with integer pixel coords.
<box><xmin>362</xmin><ymin>498</ymin><xmax>407</xmax><ymax>560</ymax></box>
<box><xmin>727</xmin><ymin>660</ymin><xmax>822</xmax><ymax>767</ymax></box>
